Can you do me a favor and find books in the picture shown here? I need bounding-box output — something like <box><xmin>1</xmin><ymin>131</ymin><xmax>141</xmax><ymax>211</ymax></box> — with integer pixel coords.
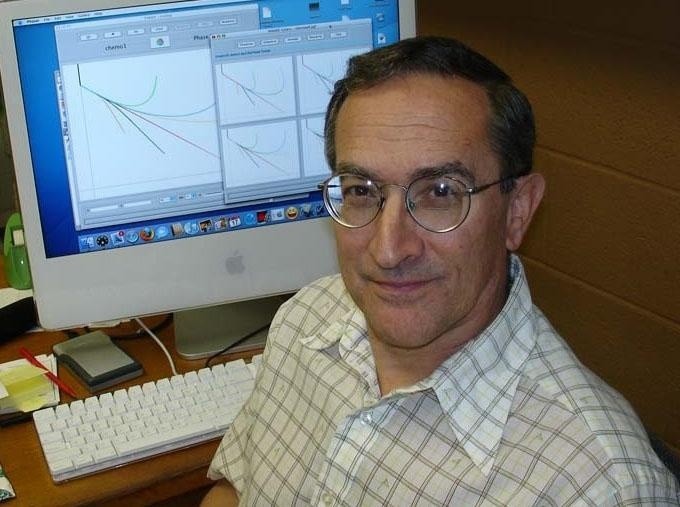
<box><xmin>1</xmin><ymin>351</ymin><xmax>62</xmax><ymax>427</ymax></box>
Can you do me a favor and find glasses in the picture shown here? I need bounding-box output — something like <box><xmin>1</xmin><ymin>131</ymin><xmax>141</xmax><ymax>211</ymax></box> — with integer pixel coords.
<box><xmin>317</xmin><ymin>172</ymin><xmax>515</xmax><ymax>234</ymax></box>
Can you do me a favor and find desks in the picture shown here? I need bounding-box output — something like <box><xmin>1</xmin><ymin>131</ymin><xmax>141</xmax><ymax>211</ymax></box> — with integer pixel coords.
<box><xmin>0</xmin><ymin>314</ymin><xmax>265</xmax><ymax>507</ymax></box>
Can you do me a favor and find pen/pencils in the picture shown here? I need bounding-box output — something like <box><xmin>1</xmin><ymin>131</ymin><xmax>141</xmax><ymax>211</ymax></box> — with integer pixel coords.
<box><xmin>18</xmin><ymin>345</ymin><xmax>77</xmax><ymax>399</ymax></box>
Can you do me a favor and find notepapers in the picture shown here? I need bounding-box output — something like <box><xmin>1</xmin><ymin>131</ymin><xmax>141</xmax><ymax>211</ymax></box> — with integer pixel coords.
<box><xmin>0</xmin><ymin>363</ymin><xmax>50</xmax><ymax>412</ymax></box>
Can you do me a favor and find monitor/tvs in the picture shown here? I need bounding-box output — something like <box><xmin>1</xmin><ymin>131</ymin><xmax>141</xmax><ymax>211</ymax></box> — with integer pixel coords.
<box><xmin>0</xmin><ymin>0</ymin><xmax>417</xmax><ymax>360</ymax></box>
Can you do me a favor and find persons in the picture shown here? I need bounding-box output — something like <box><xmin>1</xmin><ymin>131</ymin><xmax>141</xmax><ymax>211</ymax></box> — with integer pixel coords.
<box><xmin>199</xmin><ymin>34</ymin><xmax>679</xmax><ymax>507</ymax></box>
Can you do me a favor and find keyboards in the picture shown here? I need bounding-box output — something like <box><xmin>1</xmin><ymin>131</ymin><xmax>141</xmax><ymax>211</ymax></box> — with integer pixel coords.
<box><xmin>33</xmin><ymin>353</ymin><xmax>264</xmax><ymax>483</ymax></box>
